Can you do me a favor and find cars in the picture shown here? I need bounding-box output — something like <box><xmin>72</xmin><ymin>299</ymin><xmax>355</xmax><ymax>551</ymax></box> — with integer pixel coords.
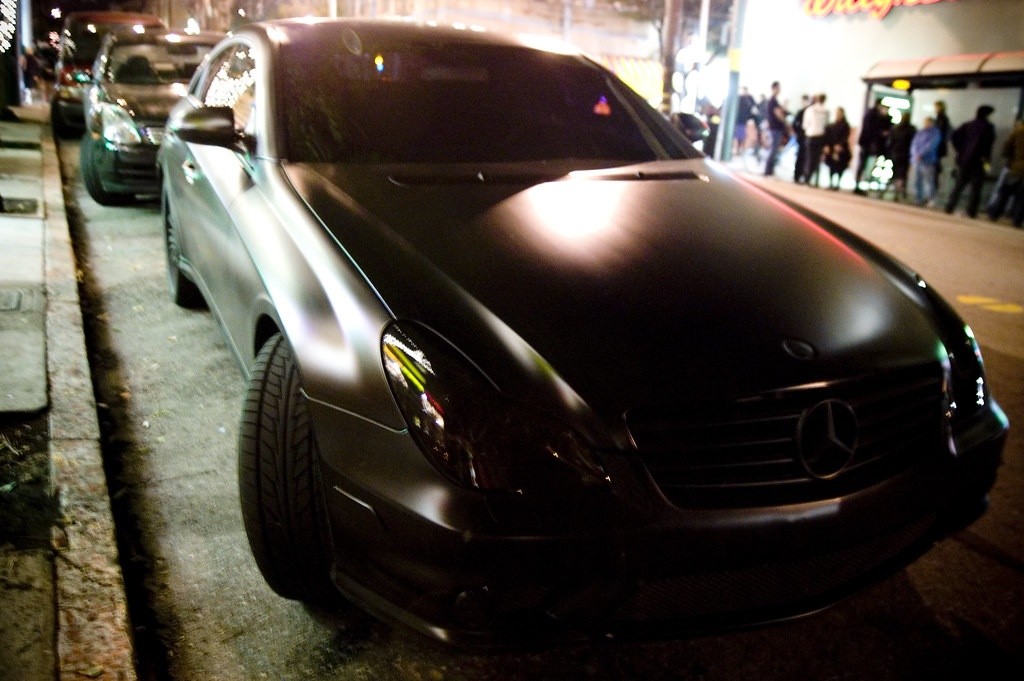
<box><xmin>74</xmin><ymin>31</ymin><xmax>231</xmax><ymax>208</ymax></box>
<box><xmin>41</xmin><ymin>12</ymin><xmax>166</xmax><ymax>140</ymax></box>
<box><xmin>156</xmin><ymin>18</ymin><xmax>1014</xmax><ymax>655</ymax></box>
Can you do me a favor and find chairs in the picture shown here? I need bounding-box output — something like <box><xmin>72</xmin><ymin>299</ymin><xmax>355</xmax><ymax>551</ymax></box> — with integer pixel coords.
<box><xmin>113</xmin><ymin>54</ymin><xmax>163</xmax><ymax>83</ymax></box>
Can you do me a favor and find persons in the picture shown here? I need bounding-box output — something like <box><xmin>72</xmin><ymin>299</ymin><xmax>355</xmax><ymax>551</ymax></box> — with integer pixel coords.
<box><xmin>19</xmin><ymin>40</ymin><xmax>57</xmax><ymax>103</ymax></box>
<box><xmin>721</xmin><ymin>78</ymin><xmax>1024</xmax><ymax>229</ymax></box>
